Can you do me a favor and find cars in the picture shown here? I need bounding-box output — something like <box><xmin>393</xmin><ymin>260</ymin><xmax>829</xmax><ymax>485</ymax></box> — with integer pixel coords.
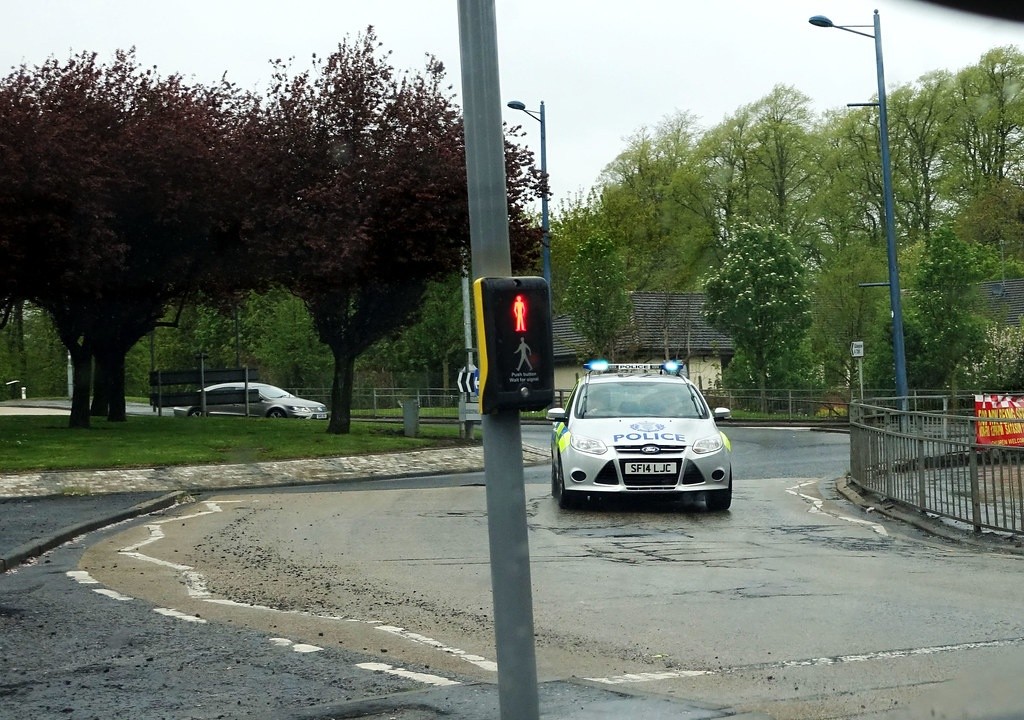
<box><xmin>174</xmin><ymin>381</ymin><xmax>330</xmax><ymax>421</ymax></box>
<box><xmin>544</xmin><ymin>360</ymin><xmax>735</xmax><ymax>510</ymax></box>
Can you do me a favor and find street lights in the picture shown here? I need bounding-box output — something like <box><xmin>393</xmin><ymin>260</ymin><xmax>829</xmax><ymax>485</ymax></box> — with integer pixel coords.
<box><xmin>504</xmin><ymin>96</ymin><xmax>555</xmax><ymax>291</ymax></box>
<box><xmin>806</xmin><ymin>10</ymin><xmax>908</xmax><ymax>421</ymax></box>
<box><xmin>194</xmin><ymin>352</ymin><xmax>210</xmax><ymax>420</ymax></box>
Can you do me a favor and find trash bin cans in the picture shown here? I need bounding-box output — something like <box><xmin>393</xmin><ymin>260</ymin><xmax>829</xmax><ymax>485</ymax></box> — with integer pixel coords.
<box><xmin>403</xmin><ymin>399</ymin><xmax>419</xmax><ymax>436</ymax></box>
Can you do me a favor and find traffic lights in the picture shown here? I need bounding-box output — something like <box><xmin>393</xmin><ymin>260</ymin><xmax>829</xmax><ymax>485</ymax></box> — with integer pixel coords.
<box><xmin>474</xmin><ymin>275</ymin><xmax>555</xmax><ymax>409</ymax></box>
